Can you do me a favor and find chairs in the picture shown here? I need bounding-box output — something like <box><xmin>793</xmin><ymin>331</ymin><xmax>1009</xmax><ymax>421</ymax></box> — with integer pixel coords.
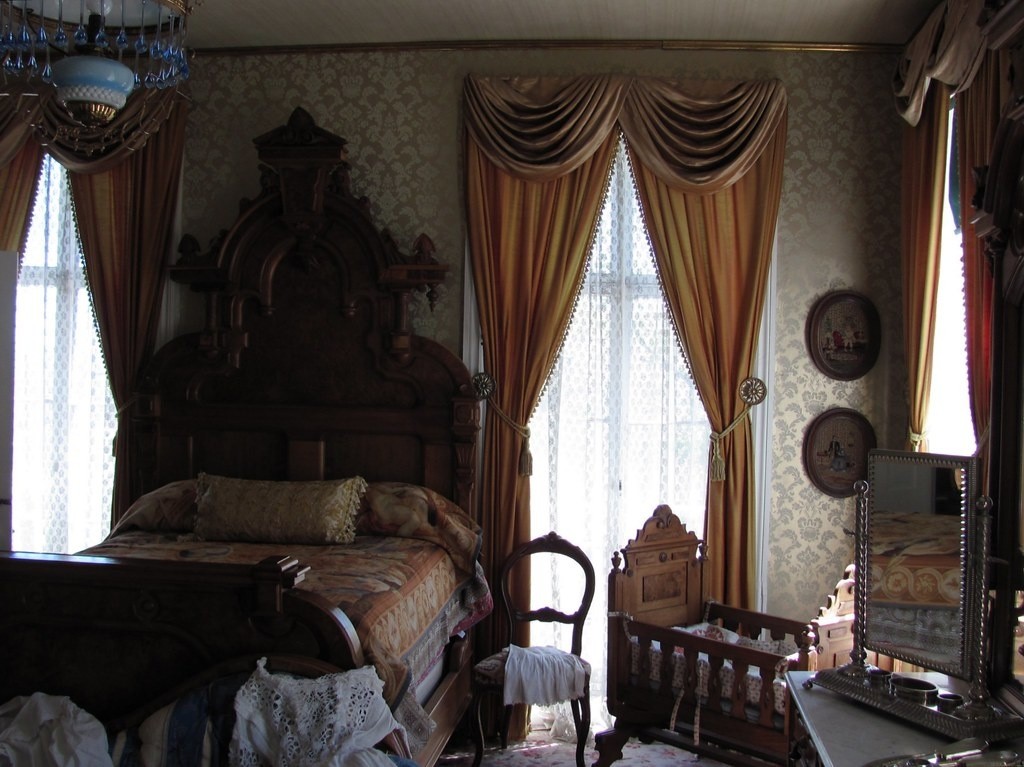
<box><xmin>470</xmin><ymin>531</ymin><xmax>590</xmax><ymax>767</ymax></box>
<box><xmin>108</xmin><ymin>653</ymin><xmax>416</xmax><ymax>767</ymax></box>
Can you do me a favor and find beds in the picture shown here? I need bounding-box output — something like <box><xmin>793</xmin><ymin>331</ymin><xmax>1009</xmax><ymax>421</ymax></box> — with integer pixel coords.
<box><xmin>592</xmin><ymin>507</ymin><xmax>893</xmax><ymax>767</ymax></box>
<box><xmin>0</xmin><ymin>105</ymin><xmax>496</xmax><ymax>767</ymax></box>
<box><xmin>866</xmin><ymin>510</ymin><xmax>963</xmax><ymax>666</ymax></box>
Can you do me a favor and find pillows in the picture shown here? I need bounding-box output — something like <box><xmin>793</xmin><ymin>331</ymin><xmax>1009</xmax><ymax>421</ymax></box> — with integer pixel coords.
<box><xmin>193</xmin><ymin>472</ymin><xmax>369</xmax><ymax>544</ymax></box>
<box><xmin>106</xmin><ymin>480</ymin><xmax>483</xmax><ymax>578</ymax></box>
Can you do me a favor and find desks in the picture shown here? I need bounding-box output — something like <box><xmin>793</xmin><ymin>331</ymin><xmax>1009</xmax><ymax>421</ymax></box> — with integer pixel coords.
<box><xmin>783</xmin><ymin>670</ymin><xmax>1024</xmax><ymax>767</ymax></box>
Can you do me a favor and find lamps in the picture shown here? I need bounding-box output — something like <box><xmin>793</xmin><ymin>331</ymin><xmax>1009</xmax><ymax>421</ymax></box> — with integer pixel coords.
<box><xmin>0</xmin><ymin>0</ymin><xmax>205</xmax><ymax>157</ymax></box>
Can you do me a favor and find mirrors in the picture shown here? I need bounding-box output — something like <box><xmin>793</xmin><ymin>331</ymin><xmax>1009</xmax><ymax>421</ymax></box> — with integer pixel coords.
<box><xmin>802</xmin><ymin>449</ymin><xmax>1024</xmax><ymax>745</ymax></box>
